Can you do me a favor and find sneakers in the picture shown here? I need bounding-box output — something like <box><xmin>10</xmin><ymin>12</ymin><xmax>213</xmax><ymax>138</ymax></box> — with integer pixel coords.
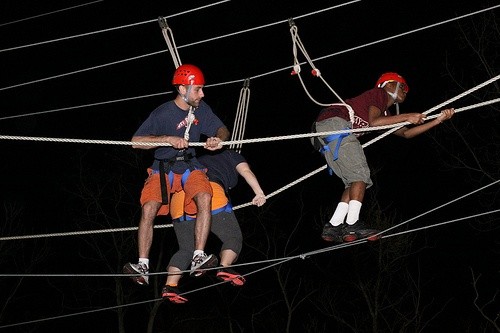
<box><xmin>190</xmin><ymin>253</ymin><xmax>218</xmax><ymax>277</ymax></box>
<box><xmin>123</xmin><ymin>262</ymin><xmax>149</xmax><ymax>285</ymax></box>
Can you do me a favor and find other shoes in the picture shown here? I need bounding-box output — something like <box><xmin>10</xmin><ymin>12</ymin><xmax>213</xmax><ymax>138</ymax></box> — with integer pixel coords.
<box><xmin>342</xmin><ymin>220</ymin><xmax>382</xmax><ymax>242</ymax></box>
<box><xmin>161</xmin><ymin>285</ymin><xmax>190</xmax><ymax>303</ymax></box>
<box><xmin>217</xmin><ymin>264</ymin><xmax>246</xmax><ymax>287</ymax></box>
<box><xmin>322</xmin><ymin>222</ymin><xmax>344</xmax><ymax>242</ymax></box>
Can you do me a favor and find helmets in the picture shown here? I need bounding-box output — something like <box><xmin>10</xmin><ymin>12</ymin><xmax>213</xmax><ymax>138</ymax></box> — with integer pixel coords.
<box><xmin>375</xmin><ymin>72</ymin><xmax>408</xmax><ymax>93</ymax></box>
<box><xmin>172</xmin><ymin>64</ymin><xmax>205</xmax><ymax>85</ymax></box>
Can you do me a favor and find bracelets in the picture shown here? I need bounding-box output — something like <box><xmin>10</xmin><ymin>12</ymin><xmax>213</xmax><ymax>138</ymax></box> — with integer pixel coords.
<box><xmin>437</xmin><ymin>118</ymin><xmax>443</xmax><ymax>123</ymax></box>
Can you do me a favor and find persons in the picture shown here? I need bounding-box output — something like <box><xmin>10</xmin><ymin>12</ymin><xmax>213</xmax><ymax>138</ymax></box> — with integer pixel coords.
<box><xmin>123</xmin><ymin>64</ymin><xmax>231</xmax><ymax>285</ymax></box>
<box><xmin>311</xmin><ymin>72</ymin><xmax>455</xmax><ymax>242</ymax></box>
<box><xmin>162</xmin><ymin>145</ymin><xmax>266</xmax><ymax>303</ymax></box>
